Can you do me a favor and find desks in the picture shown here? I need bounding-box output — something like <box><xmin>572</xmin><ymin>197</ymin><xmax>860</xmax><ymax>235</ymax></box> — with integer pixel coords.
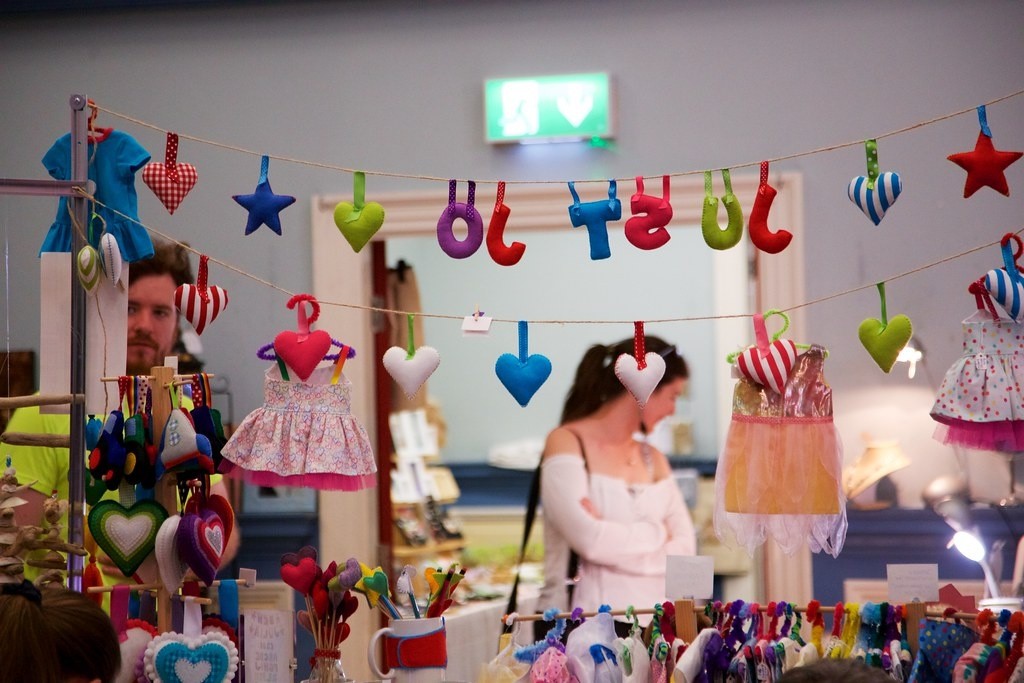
<box><xmin>390</xmin><ymin>586</ymin><xmax>541</xmax><ymax>683</ymax></box>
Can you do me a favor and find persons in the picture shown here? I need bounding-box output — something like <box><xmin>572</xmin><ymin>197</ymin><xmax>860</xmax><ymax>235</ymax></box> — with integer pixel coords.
<box><xmin>777</xmin><ymin>658</ymin><xmax>897</xmax><ymax>683</ymax></box>
<box><xmin>535</xmin><ymin>335</ymin><xmax>691</xmax><ymax>640</ymax></box>
<box><xmin>0</xmin><ymin>580</ymin><xmax>121</xmax><ymax>683</ymax></box>
<box><xmin>0</xmin><ymin>241</ymin><xmax>238</xmax><ymax>618</ymax></box>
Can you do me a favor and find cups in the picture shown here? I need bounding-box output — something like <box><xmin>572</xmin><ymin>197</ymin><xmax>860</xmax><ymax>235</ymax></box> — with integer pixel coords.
<box><xmin>367</xmin><ymin>615</ymin><xmax>447</xmax><ymax>682</ymax></box>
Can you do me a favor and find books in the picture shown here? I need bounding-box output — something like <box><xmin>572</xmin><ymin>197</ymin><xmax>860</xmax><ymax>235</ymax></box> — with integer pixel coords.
<box><xmin>390</xmin><ymin>409</ymin><xmax>457</xmax><ymax>503</ymax></box>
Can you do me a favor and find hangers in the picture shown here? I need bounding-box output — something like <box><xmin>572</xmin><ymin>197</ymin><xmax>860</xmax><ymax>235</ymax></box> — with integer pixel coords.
<box><xmin>87</xmin><ymin>98</ymin><xmax>113</xmax><ymax>142</ymax></box>
<box><xmin>255</xmin><ymin>293</ymin><xmax>355</xmax><ymax>362</ymax></box>
<box><xmin>498</xmin><ymin>595</ymin><xmax>1024</xmax><ymax>668</ymax></box>
<box><xmin>727</xmin><ymin>308</ymin><xmax>830</xmax><ymax>366</ymax></box>
<box><xmin>968</xmin><ymin>232</ymin><xmax>1024</xmax><ymax>295</ymax></box>
